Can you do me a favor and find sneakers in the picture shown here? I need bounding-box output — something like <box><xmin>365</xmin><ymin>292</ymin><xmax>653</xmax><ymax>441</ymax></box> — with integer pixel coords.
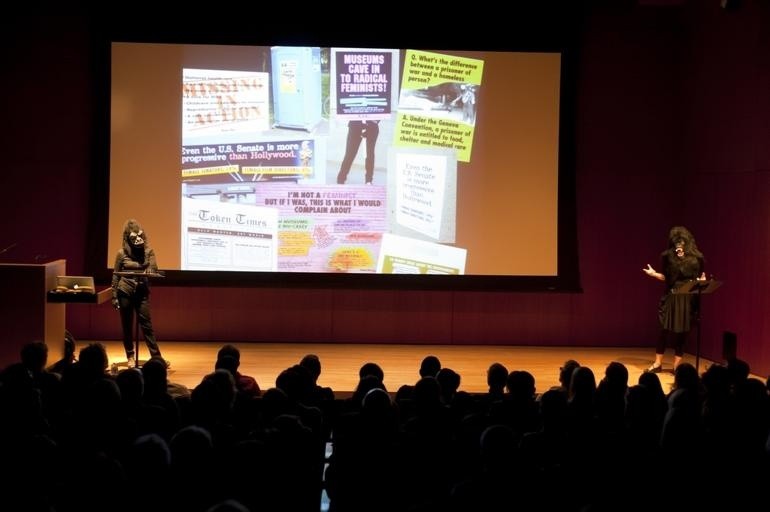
<box><xmin>128</xmin><ymin>354</ymin><xmax>136</xmax><ymax>368</ymax></box>
<box><xmin>644</xmin><ymin>364</ymin><xmax>662</xmax><ymax>373</ymax></box>
<box><xmin>164</xmin><ymin>360</ymin><xmax>171</xmax><ymax>368</ymax></box>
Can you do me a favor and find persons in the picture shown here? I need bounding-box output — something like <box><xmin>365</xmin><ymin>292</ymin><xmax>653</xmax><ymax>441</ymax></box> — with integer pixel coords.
<box><xmin>108</xmin><ymin>219</ymin><xmax>172</xmax><ymax>370</ymax></box>
<box><xmin>331</xmin><ymin>119</ymin><xmax>382</xmax><ymax>187</ymax></box>
<box><xmin>642</xmin><ymin>225</ymin><xmax>707</xmax><ymax>376</ymax></box>
<box><xmin>0</xmin><ymin>323</ymin><xmax>769</xmax><ymax>512</ymax></box>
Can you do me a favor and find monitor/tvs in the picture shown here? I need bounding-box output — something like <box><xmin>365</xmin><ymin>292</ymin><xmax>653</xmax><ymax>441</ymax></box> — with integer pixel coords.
<box><xmin>56</xmin><ymin>275</ymin><xmax>95</xmax><ymax>295</ymax></box>
